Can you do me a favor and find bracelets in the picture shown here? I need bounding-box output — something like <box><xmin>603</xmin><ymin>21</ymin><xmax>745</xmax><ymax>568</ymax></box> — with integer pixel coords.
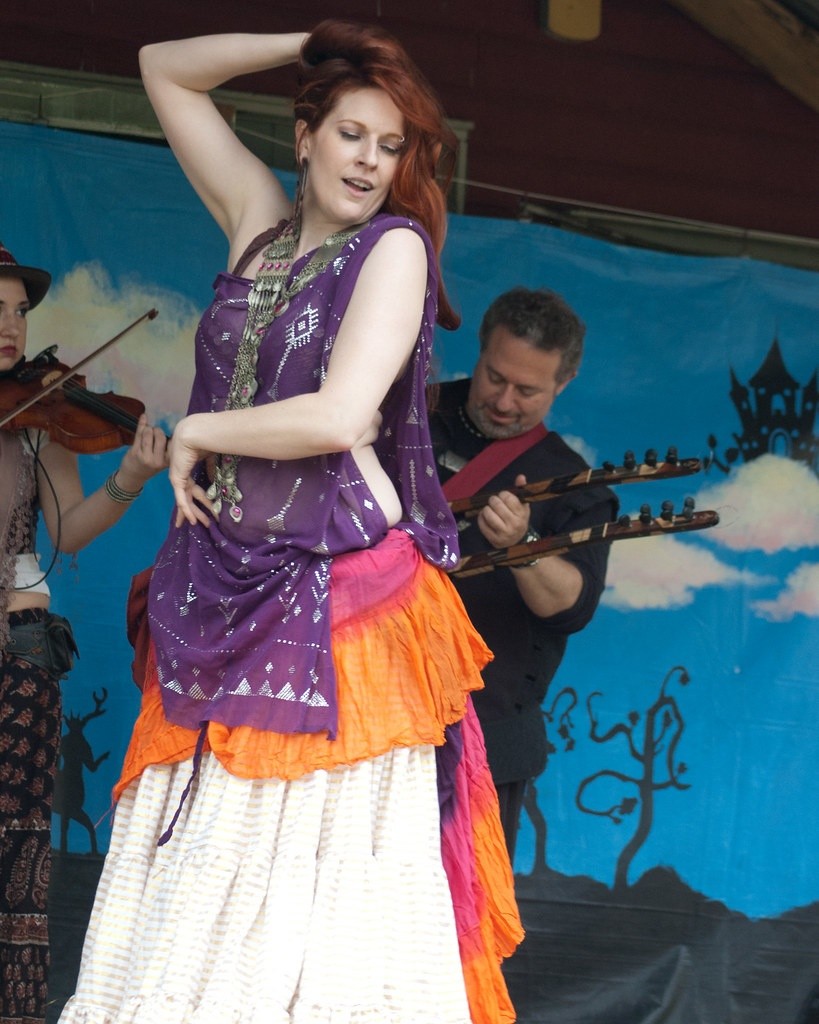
<box><xmin>518</xmin><ymin>529</ymin><xmax>539</xmax><ymax>566</ymax></box>
<box><xmin>105</xmin><ymin>469</ymin><xmax>142</xmax><ymax>504</ymax></box>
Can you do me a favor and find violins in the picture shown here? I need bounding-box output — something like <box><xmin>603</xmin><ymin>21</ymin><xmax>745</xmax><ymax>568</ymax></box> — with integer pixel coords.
<box><xmin>2</xmin><ymin>344</ymin><xmax>173</xmax><ymax>477</ymax></box>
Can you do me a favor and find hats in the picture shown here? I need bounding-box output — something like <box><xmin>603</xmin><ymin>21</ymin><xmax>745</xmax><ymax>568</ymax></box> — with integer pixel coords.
<box><xmin>0</xmin><ymin>242</ymin><xmax>51</xmax><ymax>311</ymax></box>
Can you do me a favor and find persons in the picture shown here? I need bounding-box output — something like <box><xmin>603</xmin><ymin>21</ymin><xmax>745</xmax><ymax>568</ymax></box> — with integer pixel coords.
<box><xmin>425</xmin><ymin>284</ymin><xmax>621</xmax><ymax>870</ymax></box>
<box><xmin>0</xmin><ymin>242</ymin><xmax>168</xmax><ymax>1024</ymax></box>
<box><xmin>58</xmin><ymin>28</ymin><xmax>525</xmax><ymax>1024</ymax></box>
